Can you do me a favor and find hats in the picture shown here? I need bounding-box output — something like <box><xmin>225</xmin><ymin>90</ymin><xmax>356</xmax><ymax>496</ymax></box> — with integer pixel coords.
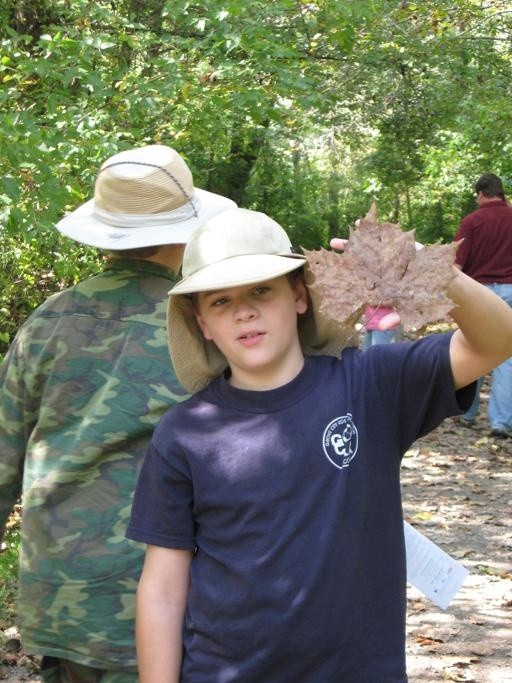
<box><xmin>54</xmin><ymin>145</ymin><xmax>238</xmax><ymax>250</ymax></box>
<box><xmin>166</xmin><ymin>208</ymin><xmax>365</xmax><ymax>395</ymax></box>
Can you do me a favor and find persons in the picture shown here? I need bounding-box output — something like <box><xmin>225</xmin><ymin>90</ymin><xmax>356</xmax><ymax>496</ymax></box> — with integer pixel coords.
<box><xmin>124</xmin><ymin>208</ymin><xmax>511</xmax><ymax>683</ymax></box>
<box><xmin>0</xmin><ymin>145</ymin><xmax>238</xmax><ymax>682</ymax></box>
<box><xmin>451</xmin><ymin>174</ymin><xmax>512</xmax><ymax>438</ymax></box>
<box><xmin>364</xmin><ymin>304</ymin><xmax>398</xmax><ymax>349</ymax></box>
